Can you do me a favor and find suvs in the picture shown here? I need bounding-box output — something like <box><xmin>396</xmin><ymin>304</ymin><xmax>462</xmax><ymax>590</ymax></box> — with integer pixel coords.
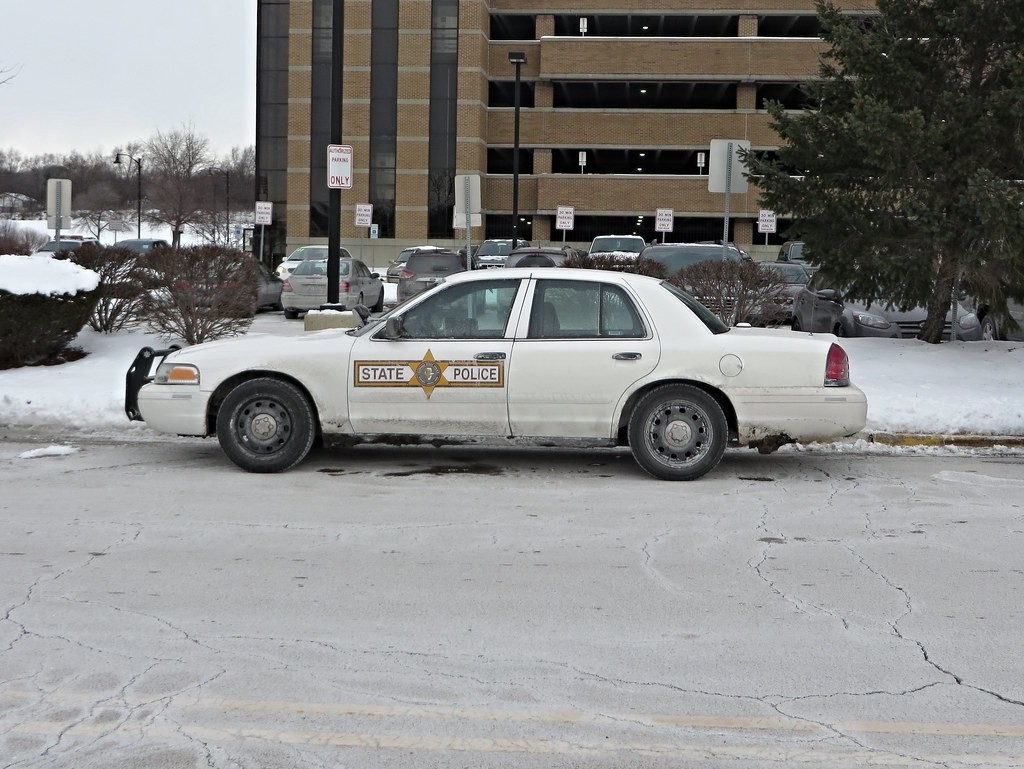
<box><xmin>635</xmin><ymin>241</ymin><xmax>750</xmax><ymax>322</ymax></box>
<box><xmin>471</xmin><ymin>238</ymin><xmax>531</xmax><ymax>271</ymax></box>
<box><xmin>395</xmin><ymin>251</ymin><xmax>466</xmax><ymax>304</ymax></box>
<box><xmin>497</xmin><ymin>242</ymin><xmax>589</xmax><ymax>316</ymax></box>
<box><xmin>775</xmin><ymin>240</ymin><xmax>826</xmax><ymax>279</ymax></box>
<box><xmin>586</xmin><ymin>235</ymin><xmax>646</xmax><ymax>270</ymax></box>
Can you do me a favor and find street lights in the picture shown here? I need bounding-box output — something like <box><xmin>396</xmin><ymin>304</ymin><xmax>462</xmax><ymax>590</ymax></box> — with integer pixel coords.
<box><xmin>506</xmin><ymin>49</ymin><xmax>529</xmax><ymax>251</ymax></box>
<box><xmin>114</xmin><ymin>154</ymin><xmax>144</xmax><ymax>238</ymax></box>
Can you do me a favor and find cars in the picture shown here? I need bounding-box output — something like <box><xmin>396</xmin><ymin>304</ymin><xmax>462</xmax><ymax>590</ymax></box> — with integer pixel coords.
<box><xmin>116</xmin><ymin>238</ymin><xmax>170</xmax><ymax>254</ymax></box>
<box><xmin>172</xmin><ymin>256</ymin><xmax>283</xmax><ymax>318</ymax></box>
<box><xmin>274</xmin><ymin>246</ymin><xmax>354</xmax><ymax>282</ymax></box>
<box><xmin>753</xmin><ymin>259</ymin><xmax>812</xmax><ymax>322</ymax></box>
<box><xmin>790</xmin><ymin>270</ymin><xmax>983</xmax><ymax>340</ymax></box>
<box><xmin>29</xmin><ymin>234</ymin><xmax>107</xmax><ymax>261</ymax></box>
<box><xmin>137</xmin><ymin>266</ymin><xmax>872</xmax><ymax>482</ymax></box>
<box><xmin>281</xmin><ymin>256</ymin><xmax>385</xmax><ymax>318</ymax></box>
<box><xmin>962</xmin><ymin>290</ymin><xmax>1024</xmax><ymax>340</ymax></box>
<box><xmin>386</xmin><ymin>245</ymin><xmax>450</xmax><ymax>282</ymax></box>
<box><xmin>456</xmin><ymin>244</ymin><xmax>482</xmax><ymax>268</ymax></box>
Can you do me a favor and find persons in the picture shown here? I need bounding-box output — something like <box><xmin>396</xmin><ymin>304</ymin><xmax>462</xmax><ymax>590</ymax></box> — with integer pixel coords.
<box><xmin>171</xmin><ymin>219</ymin><xmax>184</xmax><ymax>249</ymax></box>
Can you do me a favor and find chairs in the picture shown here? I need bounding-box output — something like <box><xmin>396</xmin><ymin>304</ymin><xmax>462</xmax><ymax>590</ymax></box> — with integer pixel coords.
<box><xmin>313</xmin><ymin>267</ymin><xmax>323</xmax><ymax>274</ymax></box>
<box><xmin>542</xmin><ymin>302</ymin><xmax>560</xmax><ymax>336</ymax></box>
